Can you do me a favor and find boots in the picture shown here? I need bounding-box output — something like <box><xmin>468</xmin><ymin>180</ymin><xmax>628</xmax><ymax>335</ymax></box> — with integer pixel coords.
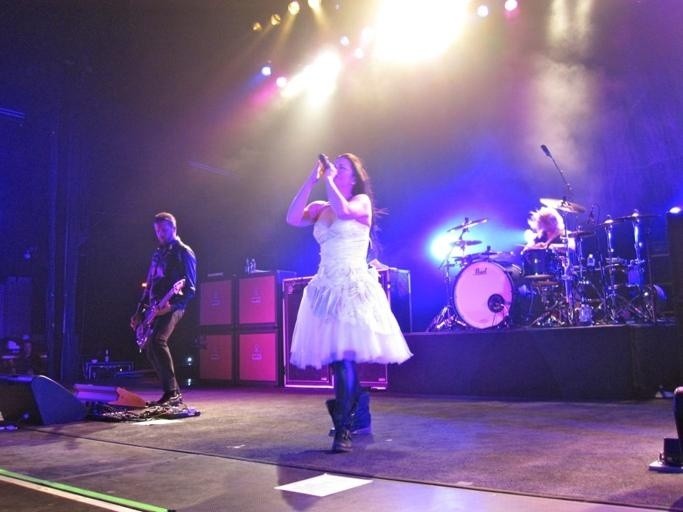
<box><xmin>329</xmin><ymin>392</ymin><xmax>371</xmax><ymax>436</ymax></box>
<box><xmin>326</xmin><ymin>399</ymin><xmax>360</xmax><ymax>453</ymax></box>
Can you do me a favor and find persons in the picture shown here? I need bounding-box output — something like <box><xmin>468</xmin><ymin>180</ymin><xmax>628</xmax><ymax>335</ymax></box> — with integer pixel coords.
<box><xmin>10</xmin><ymin>341</ymin><xmax>43</xmax><ymax>375</ymax></box>
<box><xmin>512</xmin><ymin>206</ymin><xmax>576</xmax><ymax>323</ymax></box>
<box><xmin>284</xmin><ymin>152</ymin><xmax>414</xmax><ymax>453</ymax></box>
<box><xmin>130</xmin><ymin>213</ymin><xmax>197</xmax><ymax>405</ymax></box>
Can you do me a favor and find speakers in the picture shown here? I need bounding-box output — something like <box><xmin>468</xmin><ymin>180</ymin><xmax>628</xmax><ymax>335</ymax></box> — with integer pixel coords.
<box><xmin>0</xmin><ymin>374</ymin><xmax>89</xmax><ymax>427</ymax></box>
<box><xmin>60</xmin><ymin>368</ymin><xmax>85</xmax><ymax>384</ymax></box>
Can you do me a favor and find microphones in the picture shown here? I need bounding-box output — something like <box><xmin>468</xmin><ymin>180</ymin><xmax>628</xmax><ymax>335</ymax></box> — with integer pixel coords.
<box><xmin>540</xmin><ymin>143</ymin><xmax>551</xmax><ymax>156</ymax></box>
<box><xmin>319</xmin><ymin>152</ymin><xmax>330</xmax><ymax>169</ymax></box>
<box><xmin>586</xmin><ymin>207</ymin><xmax>594</xmax><ymax>225</ymax></box>
<box><xmin>470</xmin><ymin>218</ymin><xmax>489</xmax><ymax>225</ymax></box>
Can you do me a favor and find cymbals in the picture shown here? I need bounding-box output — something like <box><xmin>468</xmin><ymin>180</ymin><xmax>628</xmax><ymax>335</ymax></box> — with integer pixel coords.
<box><xmin>445</xmin><ymin>218</ymin><xmax>487</xmax><ymax>232</ymax></box>
<box><xmin>593</xmin><ymin>218</ymin><xmax>624</xmax><ymax>228</ymax></box>
<box><xmin>614</xmin><ymin>212</ymin><xmax>659</xmax><ymax>220</ymax></box>
<box><xmin>539</xmin><ymin>198</ymin><xmax>585</xmax><ymax>213</ymax></box>
<box><xmin>562</xmin><ymin>231</ymin><xmax>594</xmax><ymax>237</ymax></box>
<box><xmin>450</xmin><ymin>240</ymin><xmax>481</xmax><ymax>246</ymax></box>
<box><xmin>452</xmin><ymin>251</ymin><xmax>517</xmax><ymax>264</ymax></box>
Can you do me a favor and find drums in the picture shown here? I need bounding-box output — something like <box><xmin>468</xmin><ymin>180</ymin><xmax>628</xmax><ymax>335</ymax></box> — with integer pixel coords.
<box><xmin>625</xmin><ymin>259</ymin><xmax>648</xmax><ymax>288</ymax></box>
<box><xmin>520</xmin><ymin>248</ymin><xmax>557</xmax><ymax>279</ymax></box>
<box><xmin>451</xmin><ymin>260</ymin><xmax>528</xmax><ymax>331</ymax></box>
<box><xmin>528</xmin><ymin>256</ymin><xmax>564</xmax><ymax>291</ymax></box>
<box><xmin>573</xmin><ymin>268</ymin><xmax>608</xmax><ymax>303</ymax></box>
<box><xmin>601</xmin><ymin>264</ymin><xmax>628</xmax><ymax>291</ymax></box>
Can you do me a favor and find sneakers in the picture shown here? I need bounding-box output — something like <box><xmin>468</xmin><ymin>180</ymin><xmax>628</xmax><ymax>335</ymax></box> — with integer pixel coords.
<box><xmin>149</xmin><ymin>393</ymin><xmax>184</xmax><ymax>407</ymax></box>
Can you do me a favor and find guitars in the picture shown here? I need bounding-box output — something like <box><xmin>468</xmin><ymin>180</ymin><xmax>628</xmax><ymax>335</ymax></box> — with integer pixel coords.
<box><xmin>136</xmin><ymin>278</ymin><xmax>186</xmax><ymax>349</ymax></box>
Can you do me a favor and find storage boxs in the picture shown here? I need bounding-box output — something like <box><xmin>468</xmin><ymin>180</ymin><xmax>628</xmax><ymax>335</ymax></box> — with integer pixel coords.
<box><xmin>196</xmin><ymin>269</ymin><xmax>296</xmax><ymax>387</ymax></box>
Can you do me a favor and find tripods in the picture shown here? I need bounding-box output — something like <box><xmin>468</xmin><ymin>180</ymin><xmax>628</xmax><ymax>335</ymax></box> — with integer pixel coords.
<box><xmin>425</xmin><ymin>229</ymin><xmax>467</xmax><ymax>332</ymax></box>
<box><xmin>529</xmin><ymin>222</ymin><xmax>658</xmax><ymax>327</ymax></box>
